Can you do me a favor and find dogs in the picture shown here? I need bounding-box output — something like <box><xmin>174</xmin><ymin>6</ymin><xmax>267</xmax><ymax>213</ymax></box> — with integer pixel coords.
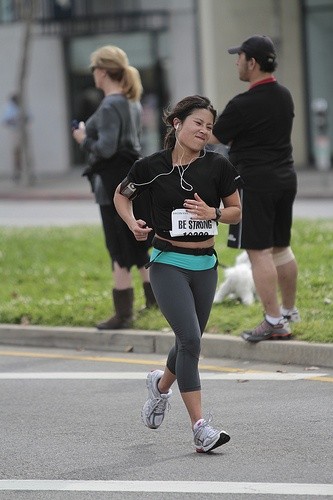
<box><xmin>211</xmin><ymin>250</ymin><xmax>256</xmax><ymax>305</ymax></box>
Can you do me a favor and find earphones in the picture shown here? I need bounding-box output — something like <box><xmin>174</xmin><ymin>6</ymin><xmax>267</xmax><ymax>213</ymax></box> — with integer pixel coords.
<box><xmin>176</xmin><ymin>123</ymin><xmax>180</xmax><ymax>130</ymax></box>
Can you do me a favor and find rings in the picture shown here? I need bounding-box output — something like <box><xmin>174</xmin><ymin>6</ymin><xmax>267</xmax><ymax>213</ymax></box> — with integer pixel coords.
<box><xmin>196</xmin><ymin>206</ymin><xmax>197</xmax><ymax>209</ymax></box>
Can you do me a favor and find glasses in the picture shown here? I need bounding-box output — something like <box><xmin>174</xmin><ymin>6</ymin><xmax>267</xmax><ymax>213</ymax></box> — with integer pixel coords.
<box><xmin>89</xmin><ymin>64</ymin><xmax>100</xmax><ymax>74</ymax></box>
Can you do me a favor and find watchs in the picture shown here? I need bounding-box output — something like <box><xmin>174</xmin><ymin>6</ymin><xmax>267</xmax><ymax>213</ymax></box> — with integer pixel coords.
<box><xmin>211</xmin><ymin>208</ymin><xmax>221</xmax><ymax>221</ymax></box>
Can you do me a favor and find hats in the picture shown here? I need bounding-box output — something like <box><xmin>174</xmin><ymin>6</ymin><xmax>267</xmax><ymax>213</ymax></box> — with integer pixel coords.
<box><xmin>228</xmin><ymin>34</ymin><xmax>277</xmax><ymax>63</ymax></box>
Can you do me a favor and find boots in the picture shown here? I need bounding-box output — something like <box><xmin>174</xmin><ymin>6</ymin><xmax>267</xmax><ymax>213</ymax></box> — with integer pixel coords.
<box><xmin>97</xmin><ymin>286</ymin><xmax>134</xmax><ymax>329</ymax></box>
<box><xmin>139</xmin><ymin>282</ymin><xmax>157</xmax><ymax>314</ymax></box>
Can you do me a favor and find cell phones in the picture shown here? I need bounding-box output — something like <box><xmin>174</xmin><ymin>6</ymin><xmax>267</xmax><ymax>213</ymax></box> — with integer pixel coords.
<box><xmin>121</xmin><ymin>185</ymin><xmax>136</xmax><ymax>199</ymax></box>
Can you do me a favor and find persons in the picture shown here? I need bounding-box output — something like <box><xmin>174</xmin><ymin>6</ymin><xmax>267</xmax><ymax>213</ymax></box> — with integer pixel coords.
<box><xmin>75</xmin><ymin>44</ymin><xmax>158</xmax><ymax>329</ymax></box>
<box><xmin>114</xmin><ymin>96</ymin><xmax>242</xmax><ymax>452</ymax></box>
<box><xmin>209</xmin><ymin>35</ymin><xmax>300</xmax><ymax>342</ymax></box>
<box><xmin>4</xmin><ymin>94</ymin><xmax>32</xmax><ymax>179</ymax></box>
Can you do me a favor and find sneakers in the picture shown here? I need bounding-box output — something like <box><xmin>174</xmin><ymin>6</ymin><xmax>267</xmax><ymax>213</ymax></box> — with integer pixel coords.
<box><xmin>141</xmin><ymin>369</ymin><xmax>174</xmax><ymax>429</ymax></box>
<box><xmin>279</xmin><ymin>304</ymin><xmax>301</xmax><ymax>323</ymax></box>
<box><xmin>242</xmin><ymin>315</ymin><xmax>292</xmax><ymax>342</ymax></box>
<box><xmin>194</xmin><ymin>409</ymin><xmax>231</xmax><ymax>452</ymax></box>
